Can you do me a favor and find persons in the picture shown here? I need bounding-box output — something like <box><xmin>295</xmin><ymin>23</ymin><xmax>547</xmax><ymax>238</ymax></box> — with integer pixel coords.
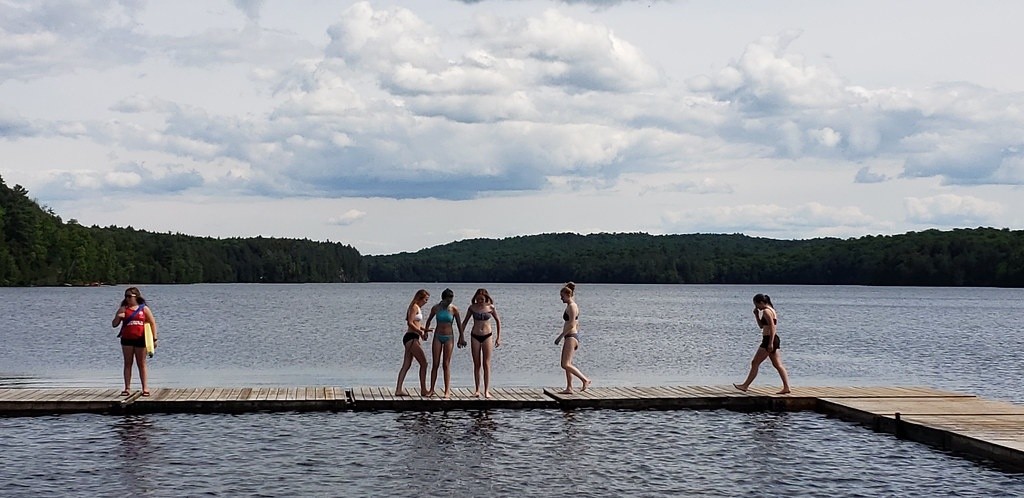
<box><xmin>424</xmin><ymin>288</ymin><xmax>467</xmax><ymax>398</ymax></box>
<box><xmin>112</xmin><ymin>286</ymin><xmax>157</xmax><ymax>397</ymax></box>
<box><xmin>395</xmin><ymin>290</ymin><xmax>434</xmax><ymax>396</ymax></box>
<box><xmin>733</xmin><ymin>294</ymin><xmax>791</xmax><ymax>394</ymax></box>
<box><xmin>555</xmin><ymin>282</ymin><xmax>591</xmax><ymax>393</ymax></box>
<box><xmin>457</xmin><ymin>288</ymin><xmax>500</xmax><ymax>398</ymax></box>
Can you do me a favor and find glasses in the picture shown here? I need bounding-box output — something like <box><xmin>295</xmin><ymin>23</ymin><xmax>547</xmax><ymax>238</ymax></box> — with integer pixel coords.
<box><xmin>125</xmin><ymin>294</ymin><xmax>136</xmax><ymax>298</ymax></box>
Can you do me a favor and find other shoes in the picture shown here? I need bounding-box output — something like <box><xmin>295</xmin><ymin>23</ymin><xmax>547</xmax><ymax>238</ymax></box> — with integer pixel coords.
<box><xmin>121</xmin><ymin>390</ymin><xmax>130</xmax><ymax>396</ymax></box>
<box><xmin>141</xmin><ymin>390</ymin><xmax>150</xmax><ymax>396</ymax></box>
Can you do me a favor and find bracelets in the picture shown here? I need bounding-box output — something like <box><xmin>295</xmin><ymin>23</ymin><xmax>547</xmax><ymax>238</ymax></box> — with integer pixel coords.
<box><xmin>153</xmin><ymin>339</ymin><xmax>157</xmax><ymax>342</ymax></box>
